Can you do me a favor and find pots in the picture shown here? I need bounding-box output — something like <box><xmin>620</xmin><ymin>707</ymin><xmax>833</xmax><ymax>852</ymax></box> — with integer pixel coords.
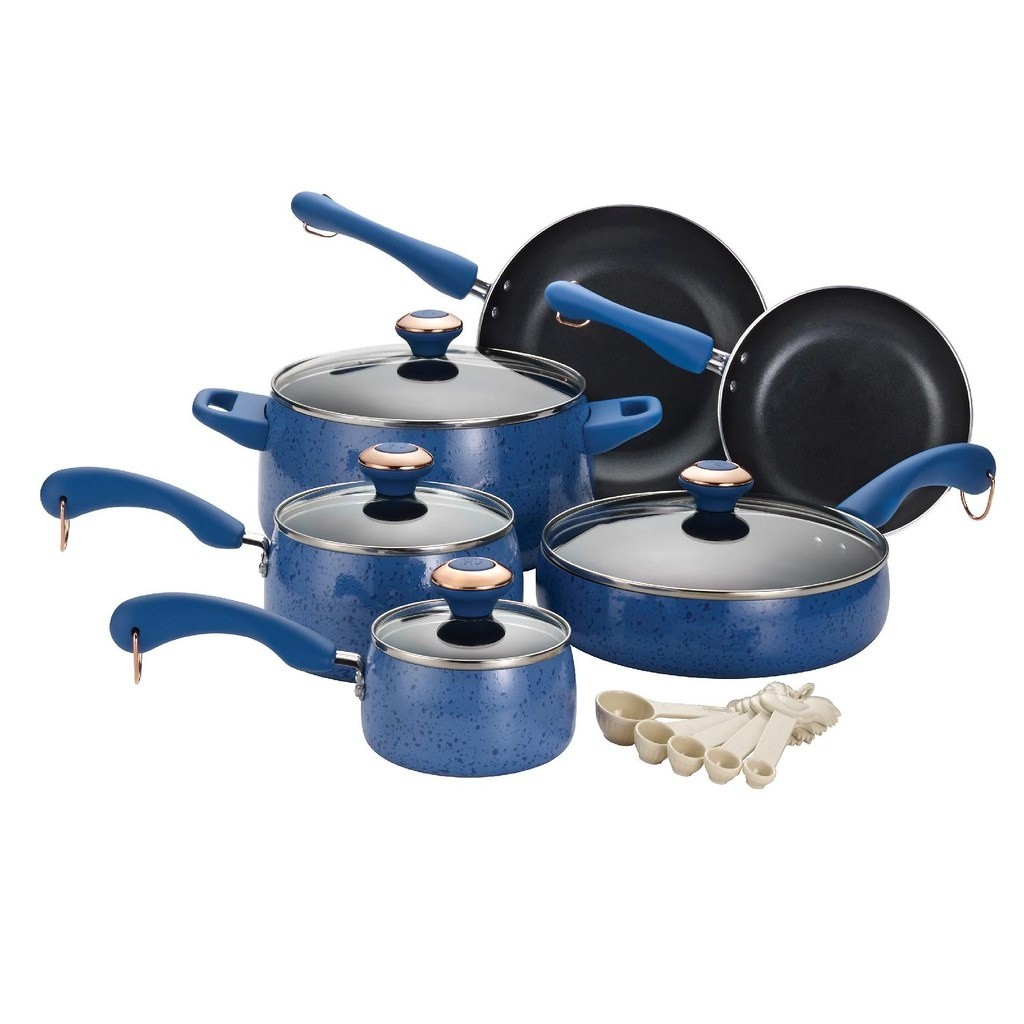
<box><xmin>41</xmin><ymin>191</ymin><xmax>995</xmax><ymax>779</ymax></box>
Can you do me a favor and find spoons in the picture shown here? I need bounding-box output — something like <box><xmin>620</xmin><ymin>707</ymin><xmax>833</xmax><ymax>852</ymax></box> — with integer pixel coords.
<box><xmin>595</xmin><ymin>682</ymin><xmax>840</xmax><ymax>789</ymax></box>
